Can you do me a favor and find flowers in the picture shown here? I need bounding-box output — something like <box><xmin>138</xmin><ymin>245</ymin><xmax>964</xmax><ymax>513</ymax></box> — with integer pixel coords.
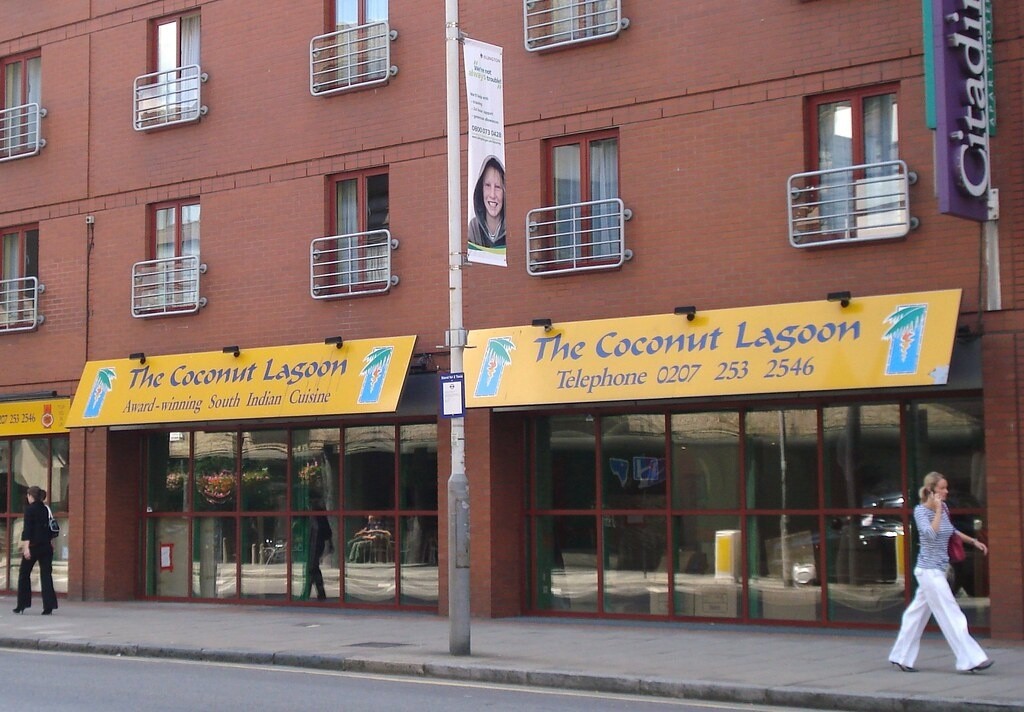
<box><xmin>296</xmin><ymin>462</ymin><xmax>322</xmax><ymax>484</ymax></box>
<box><xmin>165</xmin><ymin>466</ymin><xmax>270</xmax><ymax>505</ymax></box>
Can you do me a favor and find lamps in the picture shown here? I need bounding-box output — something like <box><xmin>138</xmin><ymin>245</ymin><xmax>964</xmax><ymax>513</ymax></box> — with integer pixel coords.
<box><xmin>674</xmin><ymin>306</ymin><xmax>696</xmax><ymax>321</ymax></box>
<box><xmin>223</xmin><ymin>346</ymin><xmax>240</xmax><ymax>357</ymax></box>
<box><xmin>325</xmin><ymin>336</ymin><xmax>343</xmax><ymax>349</ymax></box>
<box><xmin>129</xmin><ymin>352</ymin><xmax>146</xmax><ymax>365</ymax></box>
<box><xmin>532</xmin><ymin>318</ymin><xmax>552</xmax><ymax>332</ymax></box>
<box><xmin>827</xmin><ymin>291</ymin><xmax>851</xmax><ymax>307</ymax></box>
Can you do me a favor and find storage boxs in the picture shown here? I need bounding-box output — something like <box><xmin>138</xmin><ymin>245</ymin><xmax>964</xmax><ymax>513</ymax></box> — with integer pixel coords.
<box><xmin>694</xmin><ymin>585</ymin><xmax>738</xmax><ymax>617</ymax></box>
<box><xmin>761</xmin><ymin>590</ymin><xmax>817</xmax><ymax>620</ymax></box>
<box><xmin>650</xmin><ymin>591</ymin><xmax>694</xmax><ymax>616</ymax></box>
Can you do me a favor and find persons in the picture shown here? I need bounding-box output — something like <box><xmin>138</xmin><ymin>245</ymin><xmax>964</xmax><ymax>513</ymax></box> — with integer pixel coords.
<box><xmin>13</xmin><ymin>486</ymin><xmax>58</xmax><ymax>615</ymax></box>
<box><xmin>467</xmin><ymin>154</ymin><xmax>507</xmax><ymax>251</ymax></box>
<box><xmin>300</xmin><ymin>490</ymin><xmax>385</xmax><ymax>604</ymax></box>
<box><xmin>888</xmin><ymin>472</ymin><xmax>994</xmax><ymax>674</ymax></box>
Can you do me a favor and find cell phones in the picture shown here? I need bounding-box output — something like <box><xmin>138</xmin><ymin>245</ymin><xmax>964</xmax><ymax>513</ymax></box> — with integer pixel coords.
<box><xmin>929</xmin><ymin>490</ymin><xmax>935</xmax><ymax>495</ymax></box>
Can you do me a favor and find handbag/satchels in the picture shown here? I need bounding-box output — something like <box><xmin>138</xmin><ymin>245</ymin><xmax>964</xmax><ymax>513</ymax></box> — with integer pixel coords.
<box><xmin>948</xmin><ymin>533</ymin><xmax>964</xmax><ymax>561</ymax></box>
<box><xmin>45</xmin><ymin>504</ymin><xmax>60</xmax><ymax>537</ymax></box>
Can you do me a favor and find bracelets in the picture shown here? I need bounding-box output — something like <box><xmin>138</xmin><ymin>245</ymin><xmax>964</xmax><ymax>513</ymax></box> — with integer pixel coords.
<box><xmin>968</xmin><ymin>538</ymin><xmax>978</xmax><ymax>544</ymax></box>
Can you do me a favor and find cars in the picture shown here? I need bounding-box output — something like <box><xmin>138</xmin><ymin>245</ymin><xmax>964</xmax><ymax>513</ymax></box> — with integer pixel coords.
<box><xmin>775</xmin><ymin>487</ymin><xmax>1003</xmax><ymax>591</ymax></box>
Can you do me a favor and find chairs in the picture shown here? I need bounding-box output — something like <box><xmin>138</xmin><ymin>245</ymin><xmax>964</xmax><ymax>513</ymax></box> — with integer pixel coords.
<box><xmin>348</xmin><ymin>532</ymin><xmax>437</xmax><ymax>566</ymax></box>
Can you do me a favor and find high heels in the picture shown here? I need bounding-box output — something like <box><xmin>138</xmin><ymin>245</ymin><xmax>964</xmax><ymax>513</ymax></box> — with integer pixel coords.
<box><xmin>891</xmin><ymin>661</ymin><xmax>918</xmax><ymax>672</ymax></box>
<box><xmin>969</xmin><ymin>660</ymin><xmax>994</xmax><ymax>674</ymax></box>
<box><xmin>13</xmin><ymin>606</ymin><xmax>25</xmax><ymax>614</ymax></box>
<box><xmin>42</xmin><ymin>608</ymin><xmax>52</xmax><ymax>615</ymax></box>
